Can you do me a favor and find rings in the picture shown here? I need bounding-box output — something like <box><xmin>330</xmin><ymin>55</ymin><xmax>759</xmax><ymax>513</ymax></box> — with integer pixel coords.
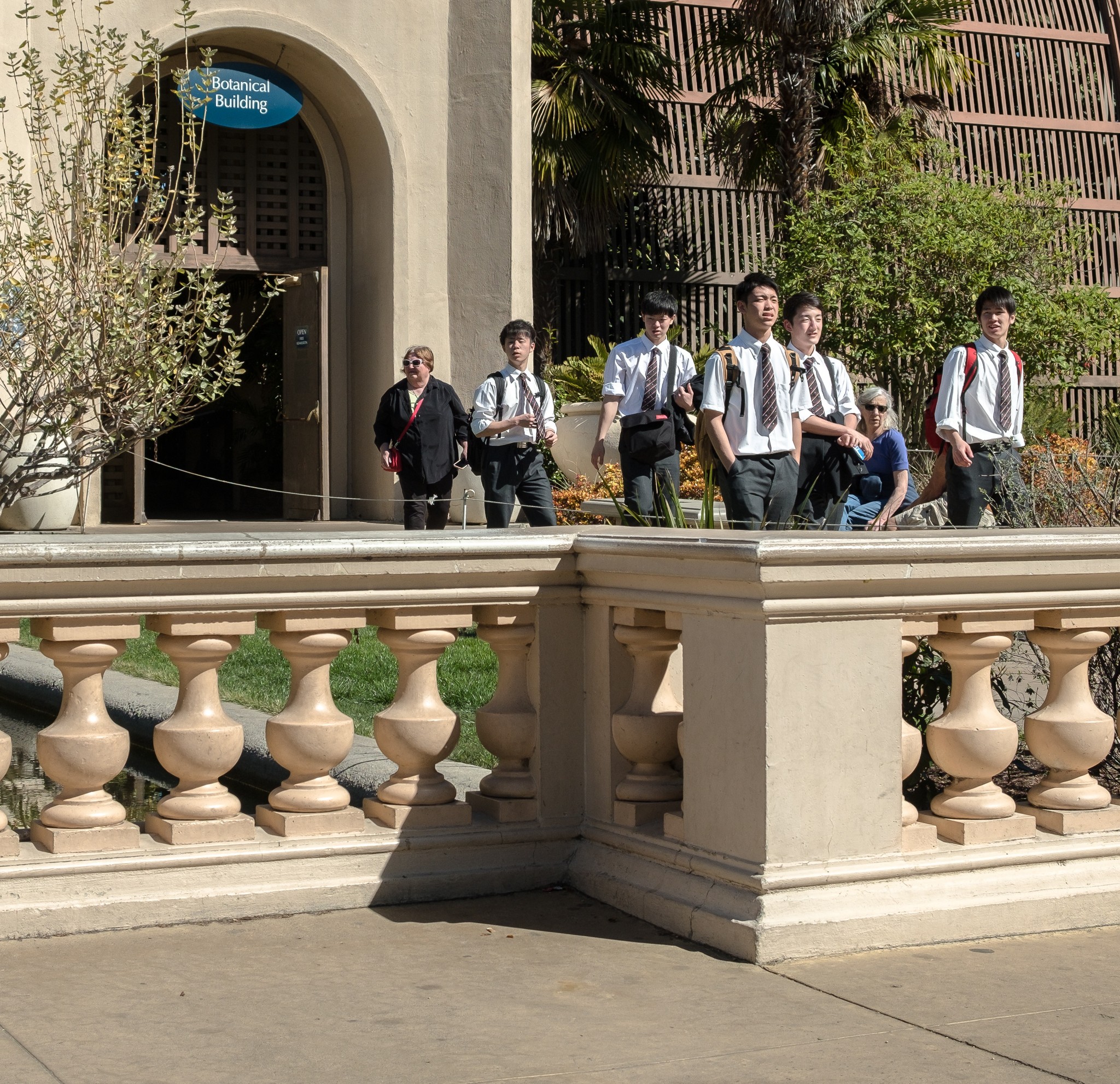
<box><xmin>380</xmin><ymin>462</ymin><xmax>384</xmax><ymax>465</ymax></box>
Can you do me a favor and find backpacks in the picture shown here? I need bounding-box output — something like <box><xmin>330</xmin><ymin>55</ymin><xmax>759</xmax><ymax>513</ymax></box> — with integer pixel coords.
<box><xmin>694</xmin><ymin>344</ymin><xmax>806</xmax><ymax>486</ymax></box>
<box><xmin>467</xmin><ymin>371</ymin><xmax>545</xmax><ymax>476</ymax></box>
<box><xmin>925</xmin><ymin>342</ymin><xmax>1022</xmax><ymax>456</ymax></box>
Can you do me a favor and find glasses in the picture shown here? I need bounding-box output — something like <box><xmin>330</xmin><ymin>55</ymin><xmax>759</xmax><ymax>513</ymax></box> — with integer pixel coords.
<box><xmin>402</xmin><ymin>359</ymin><xmax>425</xmax><ymax>367</ymax></box>
<box><xmin>864</xmin><ymin>404</ymin><xmax>888</xmax><ymax>413</ymax></box>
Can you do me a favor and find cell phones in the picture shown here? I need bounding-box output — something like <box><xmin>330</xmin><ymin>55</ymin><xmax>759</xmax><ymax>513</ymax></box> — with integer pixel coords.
<box><xmin>453</xmin><ymin>460</ymin><xmax>468</xmax><ymax>470</ymax></box>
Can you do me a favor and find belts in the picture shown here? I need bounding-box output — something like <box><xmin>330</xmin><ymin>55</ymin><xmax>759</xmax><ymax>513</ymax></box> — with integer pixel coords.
<box><xmin>509</xmin><ymin>442</ymin><xmax>530</xmax><ymax>449</ymax></box>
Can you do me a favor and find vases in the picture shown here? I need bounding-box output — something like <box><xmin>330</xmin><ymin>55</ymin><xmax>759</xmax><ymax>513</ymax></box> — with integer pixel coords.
<box><xmin>0</xmin><ymin>417</ymin><xmax>82</xmax><ymax>530</ymax></box>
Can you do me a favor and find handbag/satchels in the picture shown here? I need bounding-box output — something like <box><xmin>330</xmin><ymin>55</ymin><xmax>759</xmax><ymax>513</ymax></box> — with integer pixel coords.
<box><xmin>383</xmin><ymin>447</ymin><xmax>401</xmax><ymax>472</ymax></box>
<box><xmin>620</xmin><ymin>409</ymin><xmax>676</xmax><ymax>463</ymax></box>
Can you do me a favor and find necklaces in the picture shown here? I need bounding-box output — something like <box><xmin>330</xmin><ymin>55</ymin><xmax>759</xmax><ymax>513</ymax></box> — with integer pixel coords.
<box><xmin>411</xmin><ymin>385</ymin><xmax>420</xmax><ymax>399</ymax></box>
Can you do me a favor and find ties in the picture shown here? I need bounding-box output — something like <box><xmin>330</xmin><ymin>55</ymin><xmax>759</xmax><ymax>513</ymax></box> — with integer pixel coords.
<box><xmin>761</xmin><ymin>343</ymin><xmax>777</xmax><ymax>431</ymax></box>
<box><xmin>520</xmin><ymin>372</ymin><xmax>545</xmax><ymax>439</ymax></box>
<box><xmin>803</xmin><ymin>357</ymin><xmax>826</xmax><ymax>419</ymax></box>
<box><xmin>999</xmin><ymin>350</ymin><xmax>1011</xmax><ymax>431</ymax></box>
<box><xmin>641</xmin><ymin>348</ymin><xmax>659</xmax><ymax>411</ymax></box>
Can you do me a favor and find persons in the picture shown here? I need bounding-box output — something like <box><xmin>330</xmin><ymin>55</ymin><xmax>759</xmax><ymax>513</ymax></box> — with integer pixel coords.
<box><xmin>471</xmin><ymin>319</ymin><xmax>558</xmax><ymax>528</ymax></box>
<box><xmin>933</xmin><ymin>283</ymin><xmax>1037</xmax><ymax>530</ymax></box>
<box><xmin>699</xmin><ymin>270</ymin><xmax>813</xmax><ymax>531</ymax></box>
<box><xmin>374</xmin><ymin>345</ymin><xmax>473</xmax><ymax>530</ymax></box>
<box><xmin>591</xmin><ymin>291</ymin><xmax>697</xmax><ymax>527</ymax></box>
<box><xmin>781</xmin><ymin>291</ymin><xmax>874</xmax><ymax>531</ymax></box>
<box><xmin>838</xmin><ymin>385</ymin><xmax>920</xmax><ymax>531</ymax></box>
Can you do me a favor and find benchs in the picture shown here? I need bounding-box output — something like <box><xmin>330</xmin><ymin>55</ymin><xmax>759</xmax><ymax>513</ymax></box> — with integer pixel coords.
<box><xmin>580</xmin><ymin>499</ymin><xmax>727</xmax><ymax>524</ymax></box>
